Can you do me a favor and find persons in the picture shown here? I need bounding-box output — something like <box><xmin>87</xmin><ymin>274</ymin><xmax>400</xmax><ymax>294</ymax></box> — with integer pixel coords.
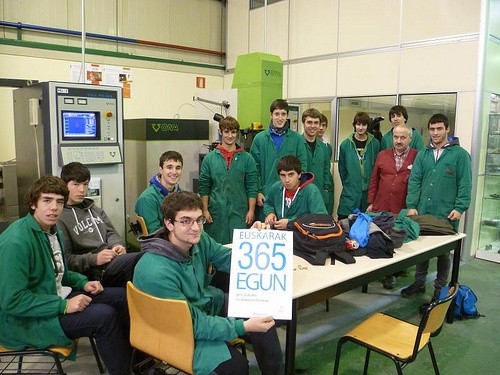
<box><xmin>133</xmin><ymin>191</ymin><xmax>285</xmax><ymax>375</ymax></box>
<box><xmin>263</xmin><ymin>155</ymin><xmax>328</xmax><ymax>230</ymax></box>
<box><xmin>198</xmin><ymin>117</ymin><xmax>258</xmax><ymax>246</ymax></box>
<box><xmin>337</xmin><ymin>112</ymin><xmax>381</xmax><ymax>225</ymax></box>
<box><xmin>401</xmin><ymin>114</ymin><xmax>472</xmax><ymax>297</ymax></box>
<box><xmin>380</xmin><ymin>106</ymin><xmax>425</xmax><ymax>277</ymax></box>
<box><xmin>51</xmin><ymin>162</ymin><xmax>142</xmax><ymax>286</ymax></box>
<box><xmin>301</xmin><ymin>108</ymin><xmax>330</xmax><ymax>214</ymax></box>
<box><xmin>366</xmin><ymin>124</ymin><xmax>419</xmax><ymax>289</ymax></box>
<box><xmin>134</xmin><ymin>150</ymin><xmax>186</xmax><ymax>237</ymax></box>
<box><xmin>249</xmin><ymin>99</ymin><xmax>307</xmax><ymax>224</ymax></box>
<box><xmin>315</xmin><ymin>114</ymin><xmax>332</xmax><ymax>167</ymax></box>
<box><xmin>0</xmin><ymin>175</ymin><xmax>144</xmax><ymax>375</ymax></box>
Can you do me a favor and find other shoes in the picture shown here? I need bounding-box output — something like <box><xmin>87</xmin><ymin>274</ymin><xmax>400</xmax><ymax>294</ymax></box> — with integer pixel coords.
<box><xmin>400</xmin><ymin>284</ymin><xmax>426</xmax><ymax>297</ymax></box>
<box><xmin>384</xmin><ymin>275</ymin><xmax>397</xmax><ymax>288</ymax></box>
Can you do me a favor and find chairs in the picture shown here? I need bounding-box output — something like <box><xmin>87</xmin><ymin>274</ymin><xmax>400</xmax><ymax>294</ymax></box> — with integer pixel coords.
<box><xmin>0</xmin><ymin>212</ymin><xmax>247</xmax><ymax>375</ymax></box>
<box><xmin>333</xmin><ymin>282</ymin><xmax>459</xmax><ymax>375</ymax></box>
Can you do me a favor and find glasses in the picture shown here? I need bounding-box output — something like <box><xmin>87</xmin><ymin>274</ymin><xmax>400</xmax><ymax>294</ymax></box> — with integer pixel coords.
<box><xmin>169</xmin><ymin>215</ymin><xmax>207</xmax><ymax>226</ymax></box>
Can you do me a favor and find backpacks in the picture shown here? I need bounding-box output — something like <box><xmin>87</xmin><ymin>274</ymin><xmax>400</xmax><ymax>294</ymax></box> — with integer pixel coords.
<box><xmin>286</xmin><ymin>213</ymin><xmax>355</xmax><ymax>265</ymax></box>
<box><xmin>436</xmin><ymin>284</ymin><xmax>478</xmax><ymax>319</ymax></box>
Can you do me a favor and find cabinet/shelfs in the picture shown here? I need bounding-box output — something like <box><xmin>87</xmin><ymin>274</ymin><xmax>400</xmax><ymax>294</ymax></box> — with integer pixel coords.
<box><xmin>474</xmin><ymin>114</ymin><xmax>500</xmax><ymax>262</ymax></box>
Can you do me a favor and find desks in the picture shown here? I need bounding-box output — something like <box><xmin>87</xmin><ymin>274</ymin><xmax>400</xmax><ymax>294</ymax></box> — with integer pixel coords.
<box><xmin>221</xmin><ymin>230</ymin><xmax>468</xmax><ymax>375</ymax></box>
